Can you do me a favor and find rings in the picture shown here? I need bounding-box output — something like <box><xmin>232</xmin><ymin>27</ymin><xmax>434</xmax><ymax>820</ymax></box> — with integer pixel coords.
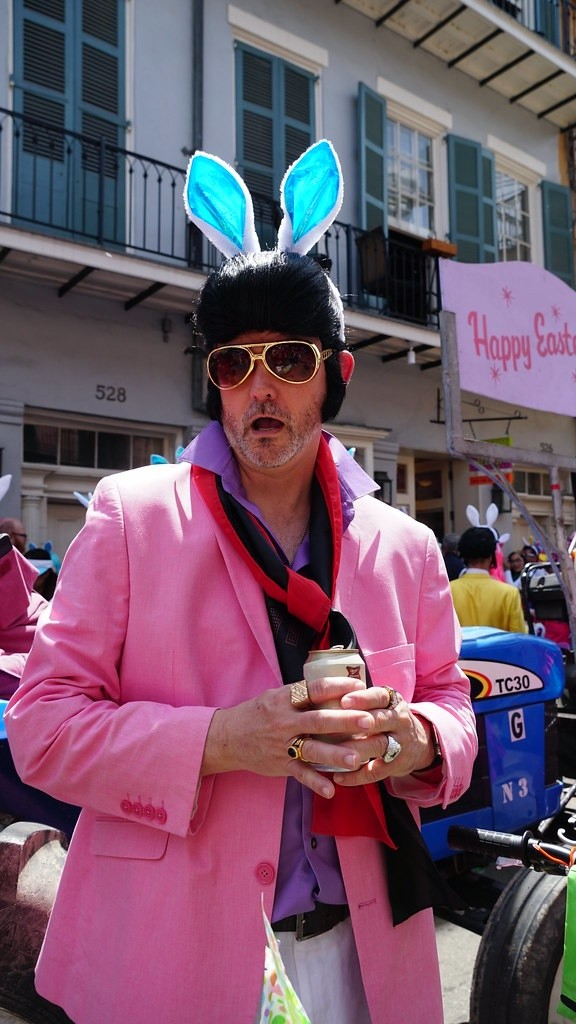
<box><xmin>383</xmin><ymin>686</ymin><xmax>398</xmax><ymax>710</ymax></box>
<box><xmin>290</xmin><ymin>680</ymin><xmax>312</xmax><ymax>707</ymax></box>
<box><xmin>382</xmin><ymin>733</ymin><xmax>402</xmax><ymax>763</ymax></box>
<box><xmin>287</xmin><ymin>737</ymin><xmax>311</xmax><ymax>762</ymax></box>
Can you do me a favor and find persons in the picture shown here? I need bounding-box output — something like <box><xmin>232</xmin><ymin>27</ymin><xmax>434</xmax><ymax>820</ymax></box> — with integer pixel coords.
<box><xmin>438</xmin><ymin>524</ymin><xmax>576</xmax><ymax>645</ymax></box>
<box><xmin>0</xmin><ymin>518</ymin><xmax>86</xmax><ymax>836</ymax></box>
<box><xmin>5</xmin><ymin>252</ymin><xmax>478</xmax><ymax>1024</ymax></box>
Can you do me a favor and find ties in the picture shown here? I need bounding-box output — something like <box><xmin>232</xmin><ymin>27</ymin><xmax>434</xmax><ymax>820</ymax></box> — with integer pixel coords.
<box><xmin>196</xmin><ymin>433</ymin><xmax>397</xmax><ymax>851</ymax></box>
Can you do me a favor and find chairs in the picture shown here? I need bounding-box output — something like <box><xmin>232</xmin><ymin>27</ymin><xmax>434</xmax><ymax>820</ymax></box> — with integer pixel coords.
<box><xmin>356</xmin><ymin>226</ymin><xmax>426</xmax><ymax>322</ymax></box>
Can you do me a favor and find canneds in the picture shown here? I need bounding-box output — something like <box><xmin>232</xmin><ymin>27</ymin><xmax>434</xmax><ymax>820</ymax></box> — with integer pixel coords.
<box><xmin>302</xmin><ymin>645</ymin><xmax>371</xmax><ymax>772</ymax></box>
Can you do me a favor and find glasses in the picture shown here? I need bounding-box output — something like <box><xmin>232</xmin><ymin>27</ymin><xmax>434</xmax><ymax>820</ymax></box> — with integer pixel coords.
<box><xmin>206</xmin><ymin>341</ymin><xmax>334</xmax><ymax>390</ymax></box>
<box><xmin>14</xmin><ymin>533</ymin><xmax>27</xmax><ymax>538</ymax></box>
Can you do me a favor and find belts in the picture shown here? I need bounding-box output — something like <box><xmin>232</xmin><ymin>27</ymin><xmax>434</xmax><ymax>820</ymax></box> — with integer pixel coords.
<box><xmin>271</xmin><ymin>901</ymin><xmax>350</xmax><ymax>941</ymax></box>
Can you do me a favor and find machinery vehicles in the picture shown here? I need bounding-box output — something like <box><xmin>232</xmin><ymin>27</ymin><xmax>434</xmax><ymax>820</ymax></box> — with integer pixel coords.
<box><xmin>0</xmin><ymin>627</ymin><xmax>576</xmax><ymax>1024</ymax></box>
